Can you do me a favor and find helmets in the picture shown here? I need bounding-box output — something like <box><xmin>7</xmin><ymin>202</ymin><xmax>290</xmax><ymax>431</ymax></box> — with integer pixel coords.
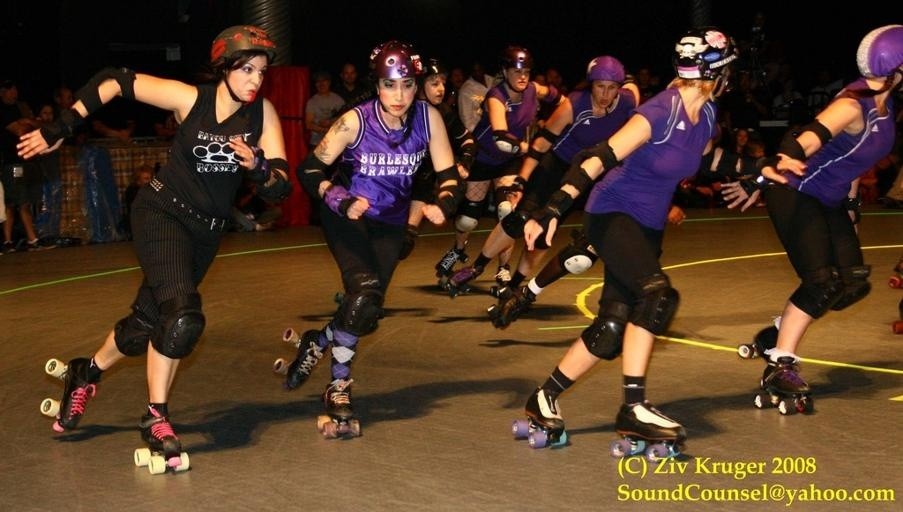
<box><xmin>854</xmin><ymin>23</ymin><xmax>903</xmax><ymax>81</ymax></box>
<box><xmin>497</xmin><ymin>46</ymin><xmax>536</xmax><ymax>73</ymax></box>
<box><xmin>672</xmin><ymin>27</ymin><xmax>742</xmax><ymax>82</ymax></box>
<box><xmin>585</xmin><ymin>55</ymin><xmax>626</xmax><ymax>83</ymax></box>
<box><xmin>365</xmin><ymin>40</ymin><xmax>428</xmax><ymax>80</ymax></box>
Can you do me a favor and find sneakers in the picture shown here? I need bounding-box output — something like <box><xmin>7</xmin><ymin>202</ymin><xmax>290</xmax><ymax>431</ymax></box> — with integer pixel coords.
<box><xmin>27</xmin><ymin>237</ymin><xmax>59</xmax><ymax>253</ymax></box>
<box><xmin>1</xmin><ymin>239</ymin><xmax>19</xmax><ymax>256</ymax></box>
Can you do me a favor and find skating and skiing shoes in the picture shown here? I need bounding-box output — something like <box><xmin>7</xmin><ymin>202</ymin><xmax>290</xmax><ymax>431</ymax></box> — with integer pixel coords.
<box><xmin>736</xmin><ymin>324</ymin><xmax>814</xmax><ymax>418</ymax></box>
<box><xmin>38</xmin><ymin>355</ymin><xmax>97</xmax><ymax>435</ymax></box>
<box><xmin>511</xmin><ymin>386</ymin><xmax>571</xmax><ymax>450</ymax></box>
<box><xmin>609</xmin><ymin>398</ymin><xmax>690</xmax><ymax>465</ymax></box>
<box><xmin>433</xmin><ymin>238</ymin><xmax>537</xmax><ymax>332</ymax></box>
<box><xmin>270</xmin><ymin>322</ymin><xmax>325</xmax><ymax>393</ymax></box>
<box><xmin>317</xmin><ymin>375</ymin><xmax>363</xmax><ymax>440</ymax></box>
<box><xmin>134</xmin><ymin>407</ymin><xmax>192</xmax><ymax>476</ymax></box>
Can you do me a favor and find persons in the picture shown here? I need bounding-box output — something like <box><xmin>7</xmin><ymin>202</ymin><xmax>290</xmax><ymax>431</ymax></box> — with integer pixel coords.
<box><xmin>0</xmin><ymin>24</ymin><xmax>903</xmax><ymax>456</ymax></box>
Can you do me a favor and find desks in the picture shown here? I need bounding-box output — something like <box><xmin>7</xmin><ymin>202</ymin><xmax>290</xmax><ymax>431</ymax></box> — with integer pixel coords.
<box><xmin>39</xmin><ymin>139</ymin><xmax>187</xmax><ymax>244</ymax></box>
